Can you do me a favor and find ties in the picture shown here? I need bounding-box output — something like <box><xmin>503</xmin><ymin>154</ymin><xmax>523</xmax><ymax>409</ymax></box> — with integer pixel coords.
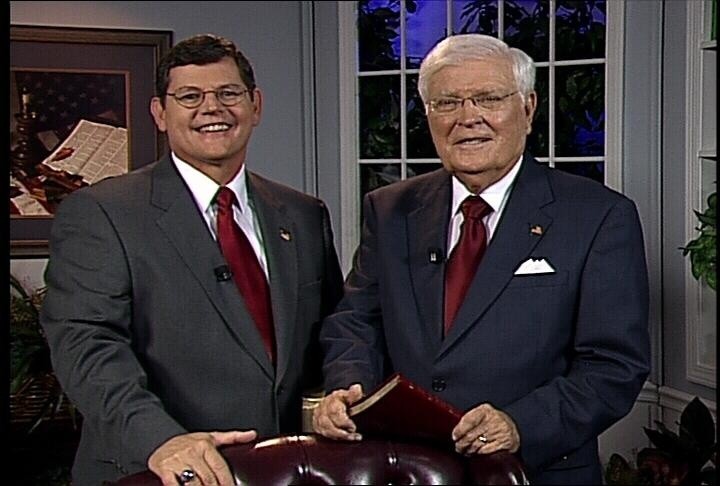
<box><xmin>443</xmin><ymin>196</ymin><xmax>494</xmax><ymax>339</ymax></box>
<box><xmin>215</xmin><ymin>186</ymin><xmax>276</xmax><ymax>361</ymax></box>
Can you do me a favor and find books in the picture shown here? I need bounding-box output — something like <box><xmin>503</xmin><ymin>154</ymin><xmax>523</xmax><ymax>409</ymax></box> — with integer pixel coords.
<box><xmin>343</xmin><ymin>370</ymin><xmax>463</xmax><ymax>449</ymax></box>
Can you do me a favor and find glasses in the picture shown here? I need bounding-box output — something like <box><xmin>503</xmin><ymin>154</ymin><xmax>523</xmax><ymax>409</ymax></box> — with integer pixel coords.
<box><xmin>165</xmin><ymin>83</ymin><xmax>252</xmax><ymax>109</ymax></box>
<box><xmin>425</xmin><ymin>90</ymin><xmax>520</xmax><ymax>113</ymax></box>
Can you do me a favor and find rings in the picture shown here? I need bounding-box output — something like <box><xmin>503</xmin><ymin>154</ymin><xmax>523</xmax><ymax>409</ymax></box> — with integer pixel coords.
<box><xmin>179</xmin><ymin>469</ymin><xmax>196</xmax><ymax>483</ymax></box>
<box><xmin>478</xmin><ymin>434</ymin><xmax>488</xmax><ymax>443</ymax></box>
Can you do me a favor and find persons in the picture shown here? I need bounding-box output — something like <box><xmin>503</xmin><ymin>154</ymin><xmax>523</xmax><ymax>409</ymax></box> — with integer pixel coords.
<box><xmin>38</xmin><ymin>34</ymin><xmax>345</xmax><ymax>486</ymax></box>
<box><xmin>311</xmin><ymin>32</ymin><xmax>653</xmax><ymax>486</ymax></box>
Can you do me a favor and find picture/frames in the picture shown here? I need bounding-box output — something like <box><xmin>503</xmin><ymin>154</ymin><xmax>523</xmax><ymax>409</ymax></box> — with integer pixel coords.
<box><xmin>11</xmin><ymin>23</ymin><xmax>175</xmax><ymax>262</ymax></box>
